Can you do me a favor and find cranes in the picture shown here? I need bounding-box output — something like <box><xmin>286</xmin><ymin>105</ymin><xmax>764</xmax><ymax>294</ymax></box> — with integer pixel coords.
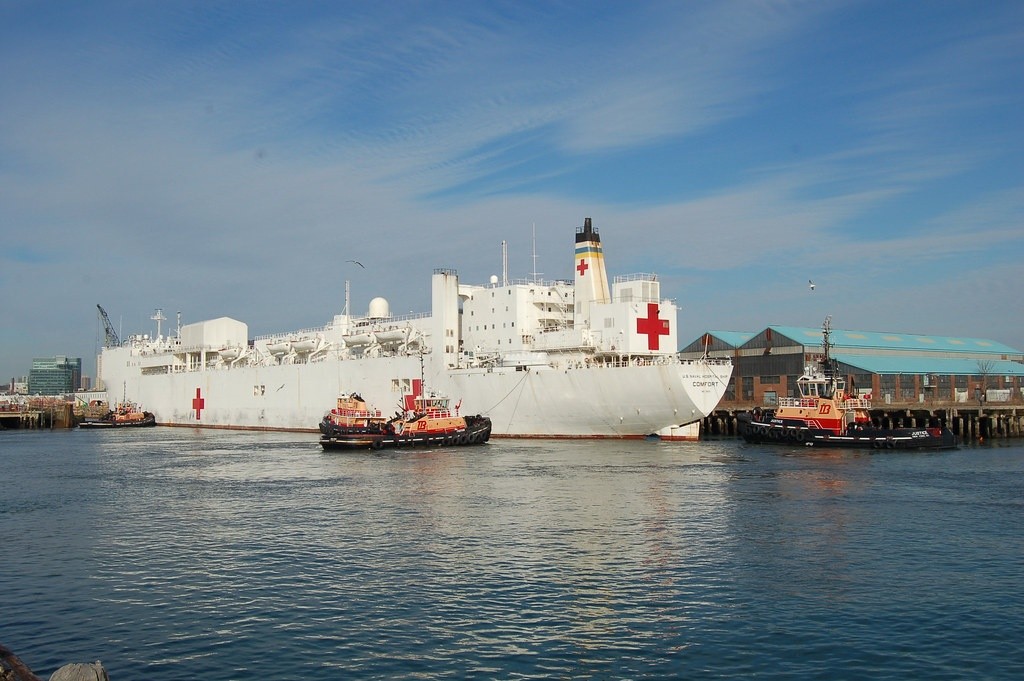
<box><xmin>97</xmin><ymin>304</ymin><xmax>120</xmax><ymax>348</ymax></box>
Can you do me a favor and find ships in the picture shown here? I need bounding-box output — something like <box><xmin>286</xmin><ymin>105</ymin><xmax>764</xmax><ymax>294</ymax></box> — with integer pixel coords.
<box><xmin>102</xmin><ymin>218</ymin><xmax>735</xmax><ymax>438</ymax></box>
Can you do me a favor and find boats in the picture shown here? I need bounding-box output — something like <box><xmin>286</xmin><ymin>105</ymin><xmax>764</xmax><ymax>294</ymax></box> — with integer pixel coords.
<box><xmin>319</xmin><ymin>347</ymin><xmax>492</xmax><ymax>451</ymax></box>
<box><xmin>736</xmin><ymin>321</ymin><xmax>958</xmax><ymax>452</ymax></box>
<box><xmin>78</xmin><ymin>382</ymin><xmax>155</xmax><ymax>428</ymax></box>
